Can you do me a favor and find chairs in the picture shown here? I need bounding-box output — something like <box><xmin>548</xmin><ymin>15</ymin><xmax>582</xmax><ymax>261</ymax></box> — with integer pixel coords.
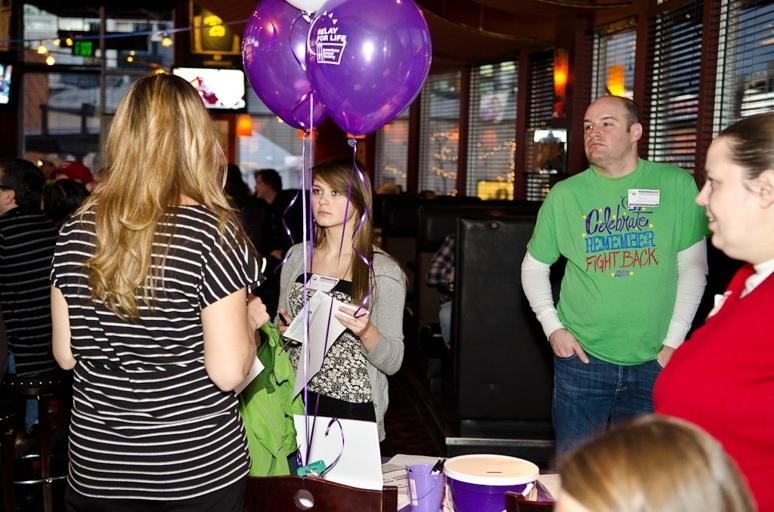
<box><xmin>444</xmin><ymin>214</ymin><xmax>567</xmax><ymax>423</ymax></box>
<box><xmin>415</xmin><ymin>209</ymin><xmax>456</xmax><ymax>363</ymax></box>
<box><xmin>245</xmin><ymin>473</ymin><xmax>399</xmax><ymax>512</ymax></box>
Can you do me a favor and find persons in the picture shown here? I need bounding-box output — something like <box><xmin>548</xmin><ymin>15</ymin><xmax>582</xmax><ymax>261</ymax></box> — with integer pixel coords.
<box><xmin>273</xmin><ymin>157</ymin><xmax>407</xmax><ymax>492</ymax></box>
<box><xmin>249</xmin><ymin>169</ymin><xmax>308</xmax><ymax>269</ymax></box>
<box><xmin>0</xmin><ymin>156</ymin><xmax>70</xmax><ymax>433</ymax></box>
<box><xmin>654</xmin><ymin>113</ymin><xmax>774</xmax><ymax>511</ymax></box>
<box><xmin>44</xmin><ymin>177</ymin><xmax>89</xmax><ymax>223</ymax></box>
<box><xmin>553</xmin><ymin>411</ymin><xmax>759</xmax><ymax>512</ymax></box>
<box><xmin>520</xmin><ymin>95</ymin><xmax>711</xmax><ymax>464</ymax></box>
<box><xmin>221</xmin><ymin>163</ymin><xmax>253</xmax><ymax>223</ymax></box>
<box><xmin>424</xmin><ymin>209</ymin><xmax>505</xmax><ymax>347</ymax></box>
<box><xmin>51</xmin><ymin>73</ymin><xmax>271</xmax><ymax>512</ymax></box>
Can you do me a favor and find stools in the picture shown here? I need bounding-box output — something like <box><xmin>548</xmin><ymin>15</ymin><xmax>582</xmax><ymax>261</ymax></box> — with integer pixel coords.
<box><xmin>0</xmin><ymin>376</ymin><xmax>72</xmax><ymax>512</ymax></box>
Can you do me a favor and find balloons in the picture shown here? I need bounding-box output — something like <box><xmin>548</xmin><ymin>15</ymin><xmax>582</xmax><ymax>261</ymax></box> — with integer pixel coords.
<box><xmin>241</xmin><ymin>0</ymin><xmax>327</xmax><ymax>131</ymax></box>
<box><xmin>307</xmin><ymin>0</ymin><xmax>432</xmax><ymax>141</ymax></box>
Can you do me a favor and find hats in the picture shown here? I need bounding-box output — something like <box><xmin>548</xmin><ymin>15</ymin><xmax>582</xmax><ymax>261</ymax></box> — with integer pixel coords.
<box><xmin>57</xmin><ymin>161</ymin><xmax>74</xmax><ymax>174</ymax></box>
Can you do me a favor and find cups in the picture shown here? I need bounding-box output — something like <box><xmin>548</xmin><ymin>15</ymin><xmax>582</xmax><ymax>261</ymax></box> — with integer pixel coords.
<box><xmin>406</xmin><ymin>465</ymin><xmax>445</xmax><ymax>512</ymax></box>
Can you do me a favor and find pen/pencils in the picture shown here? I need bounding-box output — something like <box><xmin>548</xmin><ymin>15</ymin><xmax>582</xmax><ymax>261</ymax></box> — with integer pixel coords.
<box><xmin>276</xmin><ymin>311</ymin><xmax>296</xmax><ymax>332</ymax></box>
<box><xmin>426</xmin><ymin>458</ymin><xmax>447</xmax><ymax>476</ymax></box>
